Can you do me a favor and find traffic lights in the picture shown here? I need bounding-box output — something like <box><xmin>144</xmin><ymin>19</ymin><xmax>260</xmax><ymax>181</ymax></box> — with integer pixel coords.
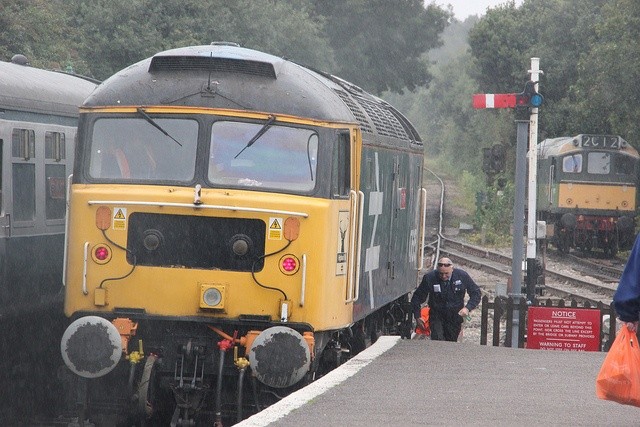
<box><xmin>515</xmin><ymin>80</ymin><xmax>544</xmax><ymax>115</ymax></box>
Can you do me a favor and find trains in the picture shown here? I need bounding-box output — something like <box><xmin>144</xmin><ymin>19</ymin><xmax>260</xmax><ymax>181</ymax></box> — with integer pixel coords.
<box><xmin>59</xmin><ymin>37</ymin><xmax>442</xmax><ymax>427</ymax></box>
<box><xmin>2</xmin><ymin>55</ymin><xmax>105</xmax><ymax>425</ymax></box>
<box><xmin>523</xmin><ymin>135</ymin><xmax>640</xmax><ymax>258</ymax></box>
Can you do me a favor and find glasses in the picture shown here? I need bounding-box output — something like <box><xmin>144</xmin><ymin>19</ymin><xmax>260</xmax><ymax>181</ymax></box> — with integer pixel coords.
<box><xmin>437</xmin><ymin>263</ymin><xmax>453</xmax><ymax>267</ymax></box>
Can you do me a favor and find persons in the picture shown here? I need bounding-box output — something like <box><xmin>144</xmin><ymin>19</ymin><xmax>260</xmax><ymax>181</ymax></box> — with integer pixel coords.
<box><xmin>612</xmin><ymin>231</ymin><xmax>639</xmax><ymax>333</ymax></box>
<box><xmin>410</xmin><ymin>257</ymin><xmax>482</xmax><ymax>342</ymax></box>
